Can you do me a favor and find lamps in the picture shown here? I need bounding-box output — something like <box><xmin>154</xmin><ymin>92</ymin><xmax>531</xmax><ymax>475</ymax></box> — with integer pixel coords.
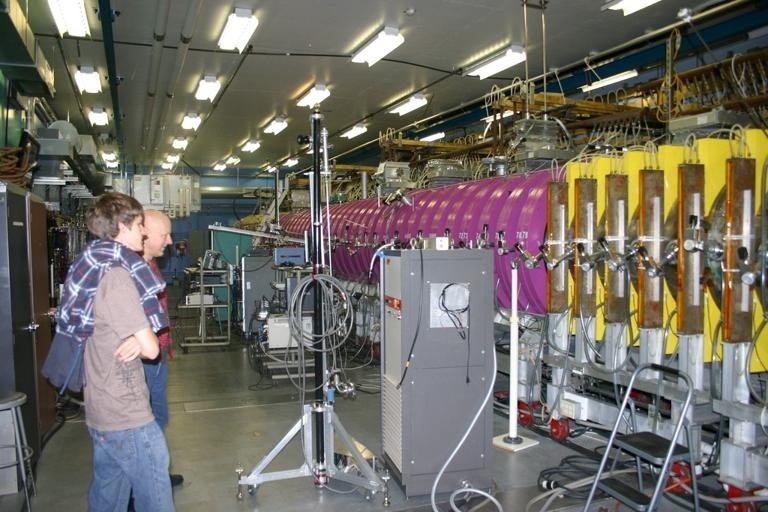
<box><xmin>171</xmin><ymin>138</ymin><xmax>189</xmax><ymax>151</ymax></box>
<box><xmin>459</xmin><ymin>41</ymin><xmax>528</xmax><ymax>81</ymax></box>
<box><xmin>213</xmin><ymin>154</ymin><xmax>242</xmax><ymax>171</ymax></box>
<box><xmin>48</xmin><ymin>0</ymin><xmax>120</xmax><ymax>170</ymax></box>
<box><xmin>282</xmin><ymin>158</ymin><xmax>300</xmax><ymax>168</ymax></box>
<box><xmin>161</xmin><ymin>155</ymin><xmax>181</xmax><ymax>172</ymax></box>
<box><xmin>387</xmin><ymin>92</ymin><xmax>428</xmax><ymax>117</ymax></box>
<box><xmin>180</xmin><ymin>112</ymin><xmax>202</xmax><ymax>132</ymax></box>
<box><xmin>294</xmin><ymin>83</ymin><xmax>332</xmax><ymax>110</ymax></box>
<box><xmin>600</xmin><ymin>0</ymin><xmax>663</xmax><ymax>15</ymax></box>
<box><xmin>349</xmin><ymin>25</ymin><xmax>406</xmax><ymax>69</ymax></box>
<box><xmin>305</xmin><ymin>144</ymin><xmax>333</xmax><ymax>155</ymax></box>
<box><xmin>216</xmin><ymin>7</ymin><xmax>260</xmax><ymax>56</ymax></box>
<box><xmin>262</xmin><ymin>116</ymin><xmax>290</xmax><ymax>136</ymax></box>
<box><xmin>418</xmin><ymin>131</ymin><xmax>447</xmax><ymax>143</ymax></box>
<box><xmin>338</xmin><ymin>123</ymin><xmax>368</xmax><ymax>140</ymax></box>
<box><xmin>263</xmin><ymin>165</ymin><xmax>279</xmax><ymax>172</ymax></box>
<box><xmin>194</xmin><ymin>75</ymin><xmax>221</xmax><ymax>104</ymax></box>
<box><xmin>240</xmin><ymin>138</ymin><xmax>262</xmax><ymax>154</ymax></box>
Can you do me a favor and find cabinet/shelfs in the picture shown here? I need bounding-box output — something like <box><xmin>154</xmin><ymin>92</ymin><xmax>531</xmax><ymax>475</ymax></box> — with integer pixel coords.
<box><xmin>1</xmin><ymin>181</ymin><xmax>57</xmax><ymax>493</ymax></box>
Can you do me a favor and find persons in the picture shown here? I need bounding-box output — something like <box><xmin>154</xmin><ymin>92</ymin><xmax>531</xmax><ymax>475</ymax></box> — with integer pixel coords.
<box><xmin>124</xmin><ymin>203</ymin><xmax>187</xmax><ymax>512</ymax></box>
<box><xmin>51</xmin><ymin>190</ymin><xmax>177</xmax><ymax>512</ymax></box>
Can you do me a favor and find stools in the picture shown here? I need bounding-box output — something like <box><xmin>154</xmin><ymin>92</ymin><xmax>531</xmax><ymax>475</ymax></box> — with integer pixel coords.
<box><xmin>0</xmin><ymin>390</ymin><xmax>36</xmax><ymax>512</ymax></box>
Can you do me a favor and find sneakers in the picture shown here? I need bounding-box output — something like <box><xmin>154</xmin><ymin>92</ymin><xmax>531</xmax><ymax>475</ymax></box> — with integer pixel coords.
<box><xmin>171</xmin><ymin>474</ymin><xmax>183</xmax><ymax>489</ymax></box>
